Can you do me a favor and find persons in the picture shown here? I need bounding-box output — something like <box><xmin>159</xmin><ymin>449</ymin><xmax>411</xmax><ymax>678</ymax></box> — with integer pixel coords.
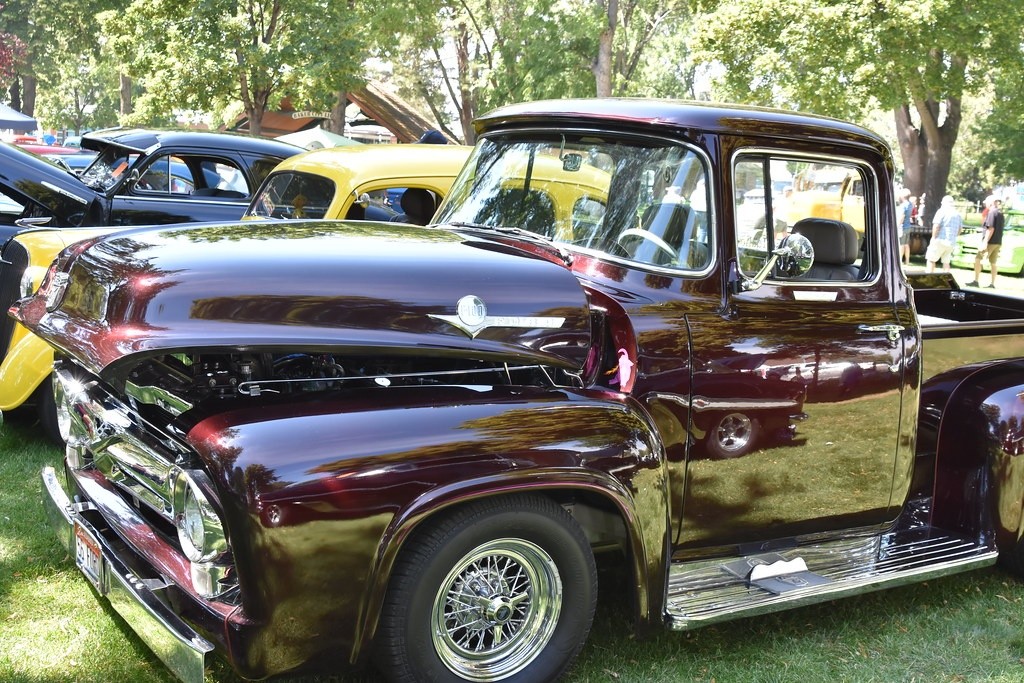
<box><xmin>895</xmin><ymin>188</ymin><xmax>926</xmax><ymax>266</ymax></box>
<box><xmin>965</xmin><ymin>196</ymin><xmax>1005</xmax><ymax>290</ymax></box>
<box><xmin>772</xmin><ymin>186</ymin><xmax>798</xmax><ymax>242</ymax></box>
<box><xmin>924</xmin><ymin>195</ymin><xmax>962</xmax><ymax>274</ymax></box>
<box><xmin>689</xmin><ymin>178</ymin><xmax>709</xmax><ymax>243</ymax></box>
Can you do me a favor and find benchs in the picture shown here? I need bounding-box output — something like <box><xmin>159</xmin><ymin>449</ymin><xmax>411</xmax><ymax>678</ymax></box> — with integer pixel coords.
<box><xmin>190</xmin><ymin>188</ymin><xmax>247</xmax><ymax>198</ymax></box>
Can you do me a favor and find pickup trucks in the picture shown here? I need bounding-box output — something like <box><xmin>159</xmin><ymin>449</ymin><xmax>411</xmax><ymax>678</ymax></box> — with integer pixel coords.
<box><xmin>8</xmin><ymin>112</ymin><xmax>1024</xmax><ymax>683</ymax></box>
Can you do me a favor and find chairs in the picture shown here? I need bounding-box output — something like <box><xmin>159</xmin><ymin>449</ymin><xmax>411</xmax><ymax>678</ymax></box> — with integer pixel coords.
<box><xmin>641</xmin><ymin>203</ymin><xmax>707</xmax><ymax>268</ymax></box>
<box><xmin>390</xmin><ymin>188</ymin><xmax>434</xmax><ymax>226</ymax></box>
<box><xmin>790</xmin><ymin>218</ymin><xmax>859</xmax><ymax>281</ymax></box>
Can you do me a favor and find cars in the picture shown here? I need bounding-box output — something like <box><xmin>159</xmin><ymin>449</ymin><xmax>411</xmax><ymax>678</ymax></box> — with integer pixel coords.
<box><xmin>949</xmin><ymin>208</ymin><xmax>1024</xmax><ymax>275</ymax></box>
<box><xmin>1</xmin><ymin>121</ymin><xmax>906</xmax><ymax>450</ymax></box>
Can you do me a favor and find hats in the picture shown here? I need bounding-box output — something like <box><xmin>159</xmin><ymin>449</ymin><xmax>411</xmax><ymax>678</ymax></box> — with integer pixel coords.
<box><xmin>982</xmin><ymin>195</ymin><xmax>997</xmax><ymax>203</ymax></box>
<box><xmin>665</xmin><ymin>187</ymin><xmax>677</xmax><ymax>192</ymax></box>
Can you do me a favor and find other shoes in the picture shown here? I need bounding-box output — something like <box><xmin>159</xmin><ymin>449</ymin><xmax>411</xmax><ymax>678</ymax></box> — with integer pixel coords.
<box><xmin>983</xmin><ymin>284</ymin><xmax>996</xmax><ymax>289</ymax></box>
<box><xmin>965</xmin><ymin>280</ymin><xmax>980</xmax><ymax>288</ymax></box>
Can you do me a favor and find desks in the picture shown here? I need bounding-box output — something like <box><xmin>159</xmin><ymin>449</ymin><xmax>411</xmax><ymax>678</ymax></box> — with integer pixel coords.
<box><xmin>16</xmin><ymin>137</ymin><xmax>37</xmax><ymax>144</ymax></box>
